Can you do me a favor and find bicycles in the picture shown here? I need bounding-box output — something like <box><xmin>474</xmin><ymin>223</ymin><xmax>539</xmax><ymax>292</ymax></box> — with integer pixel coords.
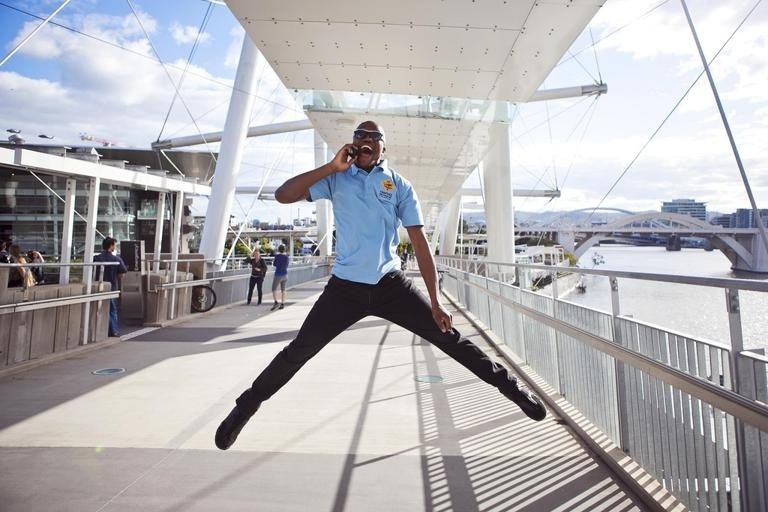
<box><xmin>188</xmin><ymin>275</ymin><xmax>219</xmax><ymax>315</ymax></box>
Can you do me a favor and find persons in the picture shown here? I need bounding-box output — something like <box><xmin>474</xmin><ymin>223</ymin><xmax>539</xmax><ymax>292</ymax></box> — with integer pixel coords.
<box><xmin>243</xmin><ymin>244</ymin><xmax>290</xmax><ymax>310</ymax></box>
<box><xmin>92</xmin><ymin>237</ymin><xmax>128</xmax><ymax>337</ymax></box>
<box><xmin>0</xmin><ymin>237</ymin><xmax>46</xmax><ymax>287</ymax></box>
<box><xmin>214</xmin><ymin>120</ymin><xmax>547</xmax><ymax>451</ymax></box>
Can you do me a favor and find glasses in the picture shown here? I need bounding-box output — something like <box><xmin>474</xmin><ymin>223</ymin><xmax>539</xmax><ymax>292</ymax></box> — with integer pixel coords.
<box><xmin>353</xmin><ymin>129</ymin><xmax>384</xmax><ymax>141</ymax></box>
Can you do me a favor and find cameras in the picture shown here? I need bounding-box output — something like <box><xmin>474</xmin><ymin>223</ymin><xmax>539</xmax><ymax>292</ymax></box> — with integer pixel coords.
<box><xmin>26</xmin><ymin>250</ymin><xmax>33</xmax><ymax>263</ymax></box>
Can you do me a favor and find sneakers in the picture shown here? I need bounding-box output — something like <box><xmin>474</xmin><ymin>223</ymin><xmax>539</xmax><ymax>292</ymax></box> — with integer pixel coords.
<box><xmin>508</xmin><ymin>381</ymin><xmax>547</xmax><ymax>422</ymax></box>
<box><xmin>270</xmin><ymin>303</ymin><xmax>284</xmax><ymax>311</ymax></box>
<box><xmin>215</xmin><ymin>404</ymin><xmax>251</xmax><ymax>450</ymax></box>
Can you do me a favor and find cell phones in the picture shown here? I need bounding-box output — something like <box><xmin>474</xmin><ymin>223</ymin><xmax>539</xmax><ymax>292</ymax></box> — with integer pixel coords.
<box><xmin>349</xmin><ymin>147</ymin><xmax>357</xmax><ymax>159</ymax></box>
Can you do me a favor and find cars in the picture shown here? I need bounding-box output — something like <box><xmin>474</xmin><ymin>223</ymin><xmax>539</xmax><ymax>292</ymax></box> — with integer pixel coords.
<box><xmin>6</xmin><ymin>231</ymin><xmax>129</xmax><ymax>260</ymax></box>
